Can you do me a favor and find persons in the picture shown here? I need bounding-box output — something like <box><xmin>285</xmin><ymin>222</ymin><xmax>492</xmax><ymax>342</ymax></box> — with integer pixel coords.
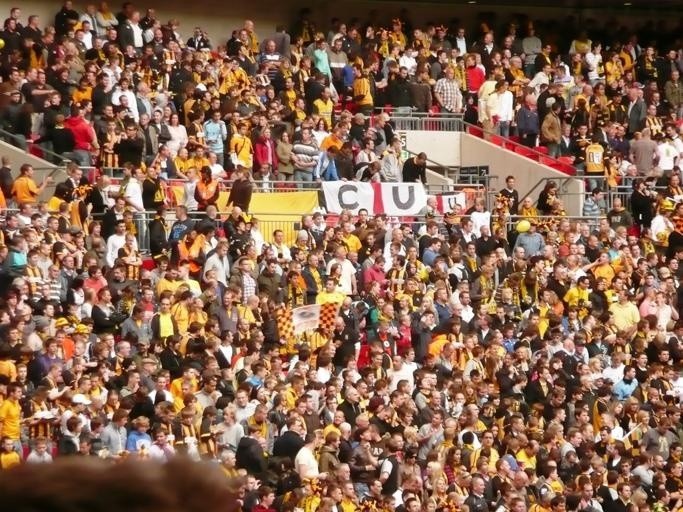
<box><xmin>0</xmin><ymin>1</ymin><xmax>683</xmax><ymax>511</ymax></box>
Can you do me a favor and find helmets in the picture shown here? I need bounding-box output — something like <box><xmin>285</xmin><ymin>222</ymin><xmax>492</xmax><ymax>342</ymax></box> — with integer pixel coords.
<box><xmin>137</xmin><ymin>337</ymin><xmax>150</xmax><ymax>345</ymax></box>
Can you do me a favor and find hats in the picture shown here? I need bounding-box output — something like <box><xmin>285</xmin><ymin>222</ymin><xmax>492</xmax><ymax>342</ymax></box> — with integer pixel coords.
<box><xmin>660</xmin><ymin>201</ymin><xmax>673</xmax><ymax>210</ymax></box>
<box><xmin>73</xmin><ymin>394</ymin><xmax>92</xmax><ymax>405</ymax></box>
<box><xmin>74</xmin><ymin>324</ymin><xmax>90</xmax><ymax>334</ymax></box>
<box><xmin>54</xmin><ymin>318</ymin><xmax>70</xmax><ymax>327</ymax></box>
<box><xmin>298</xmin><ymin>230</ymin><xmax>309</xmax><ymax>246</ymax></box>
<box><xmin>142</xmin><ymin>358</ymin><xmax>159</xmax><ymax>363</ymax></box>
<box><xmin>35</xmin><ymin>319</ymin><xmax>48</xmax><ymax>331</ymax></box>
